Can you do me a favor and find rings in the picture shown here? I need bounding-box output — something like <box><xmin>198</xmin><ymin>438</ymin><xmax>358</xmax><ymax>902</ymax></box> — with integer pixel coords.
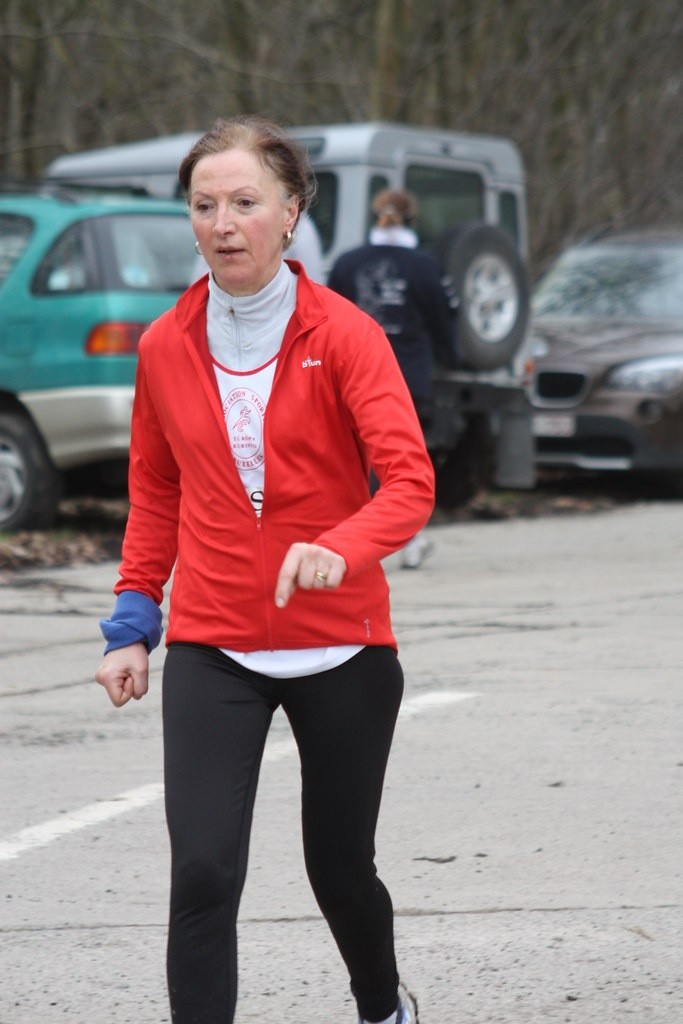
<box><xmin>315</xmin><ymin>572</ymin><xmax>327</xmax><ymax>579</ymax></box>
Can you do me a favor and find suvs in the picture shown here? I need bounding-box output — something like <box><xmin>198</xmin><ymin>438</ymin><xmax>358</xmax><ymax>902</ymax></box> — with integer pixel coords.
<box><xmin>0</xmin><ymin>181</ymin><xmax>205</xmax><ymax>537</ymax></box>
<box><xmin>43</xmin><ymin>121</ymin><xmax>546</xmax><ymax>507</ymax></box>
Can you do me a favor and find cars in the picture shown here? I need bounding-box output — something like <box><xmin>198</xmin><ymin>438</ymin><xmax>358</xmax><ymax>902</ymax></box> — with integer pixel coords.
<box><xmin>510</xmin><ymin>231</ymin><xmax>683</xmax><ymax>501</ymax></box>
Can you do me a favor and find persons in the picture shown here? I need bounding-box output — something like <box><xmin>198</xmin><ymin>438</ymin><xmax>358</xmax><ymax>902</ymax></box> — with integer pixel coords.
<box><xmin>93</xmin><ymin>113</ymin><xmax>438</xmax><ymax>1024</ymax></box>
<box><xmin>326</xmin><ymin>189</ymin><xmax>457</xmax><ymax>571</ymax></box>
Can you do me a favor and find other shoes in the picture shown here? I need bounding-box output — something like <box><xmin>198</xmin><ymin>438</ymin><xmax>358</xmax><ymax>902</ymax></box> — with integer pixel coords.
<box><xmin>402</xmin><ymin>540</ymin><xmax>431</xmax><ymax>569</ymax></box>
<box><xmin>359</xmin><ymin>981</ymin><xmax>418</xmax><ymax>1024</ymax></box>
